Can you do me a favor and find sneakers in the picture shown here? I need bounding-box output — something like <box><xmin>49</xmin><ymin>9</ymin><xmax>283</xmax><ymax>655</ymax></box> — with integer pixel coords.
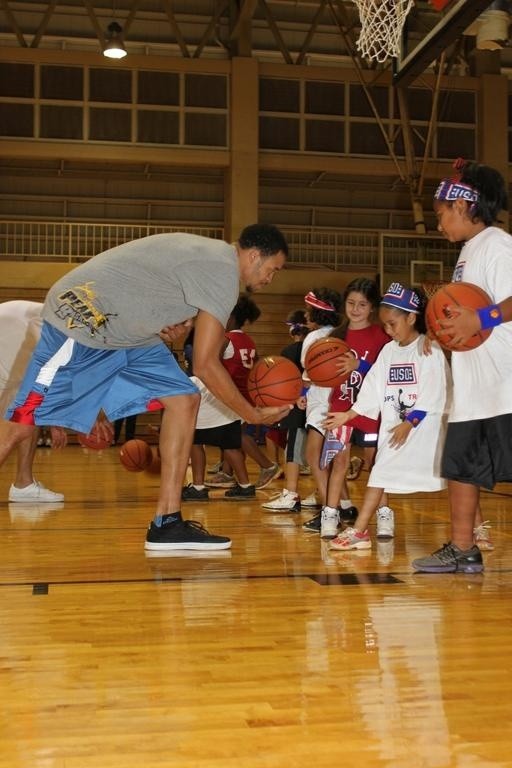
<box><xmin>300</xmin><ymin>489</ymin><xmax>325</xmax><ymax>508</ymax></box>
<box><xmin>205</xmin><ymin>473</ymin><xmax>239</xmax><ymax>489</ymax></box>
<box><xmin>208</xmin><ymin>462</ymin><xmax>225</xmax><ymax>475</ymax></box>
<box><xmin>376</xmin><ymin>506</ymin><xmax>398</xmax><ymax>539</ymax></box>
<box><xmin>470</xmin><ymin>519</ymin><xmax>495</xmax><ymax>552</ymax></box>
<box><xmin>145</xmin><ymin>517</ymin><xmax>232</xmax><ymax>550</ymax></box>
<box><xmin>8</xmin><ymin>478</ymin><xmax>65</xmax><ymax>503</ymax></box>
<box><xmin>260</xmin><ymin>488</ymin><xmax>303</xmax><ymax>516</ymax></box>
<box><xmin>223</xmin><ymin>482</ymin><xmax>258</xmax><ymax>500</ymax></box>
<box><xmin>252</xmin><ymin>462</ymin><xmax>284</xmax><ymax>490</ymax></box>
<box><xmin>346</xmin><ymin>456</ymin><xmax>366</xmax><ymax>481</ymax></box>
<box><xmin>326</xmin><ymin>527</ymin><xmax>373</xmax><ymax>553</ymax></box>
<box><xmin>341</xmin><ymin>507</ymin><xmax>359</xmax><ymax>525</ymax></box>
<box><xmin>318</xmin><ymin>506</ymin><xmax>343</xmax><ymax>540</ymax></box>
<box><xmin>181</xmin><ymin>481</ymin><xmax>211</xmax><ymax>502</ymax></box>
<box><xmin>412</xmin><ymin>540</ymin><xmax>487</xmax><ymax>576</ymax></box>
<box><xmin>300</xmin><ymin>511</ymin><xmax>322</xmax><ymax>533</ymax></box>
<box><xmin>300</xmin><ymin>465</ymin><xmax>311</xmax><ymax>477</ymax></box>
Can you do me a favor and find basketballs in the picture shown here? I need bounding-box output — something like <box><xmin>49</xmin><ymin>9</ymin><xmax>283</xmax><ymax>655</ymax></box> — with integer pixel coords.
<box><xmin>304</xmin><ymin>336</ymin><xmax>350</xmax><ymax>388</ymax></box>
<box><xmin>247</xmin><ymin>354</ymin><xmax>304</xmax><ymax>406</ymax></box>
<box><xmin>424</xmin><ymin>282</ymin><xmax>493</xmax><ymax>352</ymax></box>
<box><xmin>78</xmin><ymin>427</ymin><xmax>112</xmax><ymax>450</ymax></box>
<box><xmin>119</xmin><ymin>440</ymin><xmax>153</xmax><ymax>473</ymax></box>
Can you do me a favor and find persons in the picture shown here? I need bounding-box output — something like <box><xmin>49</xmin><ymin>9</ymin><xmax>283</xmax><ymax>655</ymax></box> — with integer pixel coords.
<box><xmin>295</xmin><ymin>286</ymin><xmax>359</xmax><ymax>533</ymax></box>
<box><xmin>320</xmin><ymin>276</ymin><xmax>396</xmax><ymax>538</ymax></box>
<box><xmin>203</xmin><ymin>296</ymin><xmax>283</xmax><ymax>490</ymax></box>
<box><xmin>319</xmin><ymin>281</ymin><xmax>496</xmax><ymax>552</ymax></box>
<box><xmin>259</xmin><ymin>308</ymin><xmax>320</xmax><ymax>514</ymax></box>
<box><xmin>0</xmin><ymin>300</ymin><xmax>115</xmax><ymax>503</ymax></box>
<box><xmin>411</xmin><ymin>156</ymin><xmax>512</xmax><ymax>575</ymax></box>
<box><xmin>181</xmin><ymin>327</ymin><xmax>257</xmax><ymax>501</ymax></box>
<box><xmin>1</xmin><ymin>223</ymin><xmax>295</xmax><ymax>552</ymax></box>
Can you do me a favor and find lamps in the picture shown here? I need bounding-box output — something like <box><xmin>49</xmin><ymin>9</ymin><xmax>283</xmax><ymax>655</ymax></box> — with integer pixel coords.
<box><xmin>103</xmin><ymin>21</ymin><xmax>127</xmax><ymax>60</ymax></box>
